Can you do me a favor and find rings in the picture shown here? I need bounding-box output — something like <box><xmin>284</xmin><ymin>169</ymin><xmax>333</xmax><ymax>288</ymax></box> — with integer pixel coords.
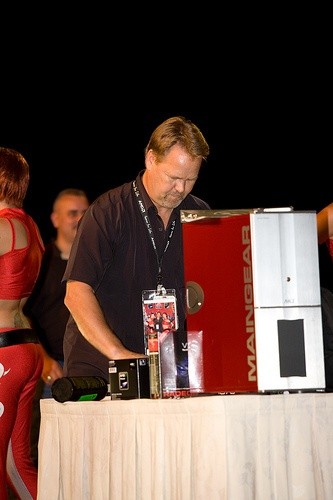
<box><xmin>47</xmin><ymin>375</ymin><xmax>51</xmax><ymax>381</ymax></box>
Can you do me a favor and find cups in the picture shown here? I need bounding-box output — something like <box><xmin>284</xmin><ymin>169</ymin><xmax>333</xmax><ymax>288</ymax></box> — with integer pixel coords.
<box><xmin>52</xmin><ymin>376</ymin><xmax>108</xmax><ymax>403</ymax></box>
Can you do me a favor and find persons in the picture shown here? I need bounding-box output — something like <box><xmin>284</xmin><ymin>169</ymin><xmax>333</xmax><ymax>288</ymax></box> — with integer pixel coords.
<box><xmin>146</xmin><ymin>312</ymin><xmax>175</xmax><ymax>338</ymax></box>
<box><xmin>317</xmin><ymin>202</ymin><xmax>333</xmax><ymax>393</ymax></box>
<box><xmin>22</xmin><ymin>189</ymin><xmax>90</xmax><ymax>470</ymax></box>
<box><xmin>62</xmin><ymin>117</ymin><xmax>210</xmax><ymax>379</ymax></box>
<box><xmin>0</xmin><ymin>147</ymin><xmax>44</xmax><ymax>500</ymax></box>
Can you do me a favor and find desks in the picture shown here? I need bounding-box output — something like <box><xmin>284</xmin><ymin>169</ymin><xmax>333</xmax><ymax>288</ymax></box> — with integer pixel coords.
<box><xmin>38</xmin><ymin>390</ymin><xmax>333</xmax><ymax>500</ymax></box>
